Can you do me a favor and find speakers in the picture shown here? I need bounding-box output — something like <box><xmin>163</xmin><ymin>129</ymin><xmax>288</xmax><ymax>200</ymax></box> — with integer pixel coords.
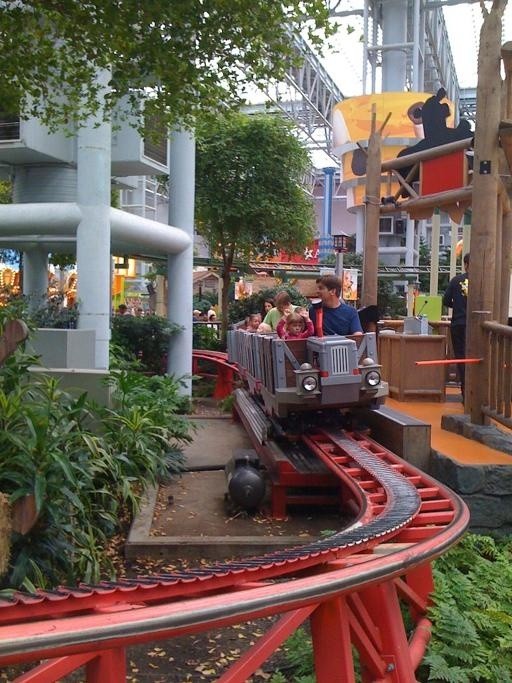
<box><xmin>396</xmin><ymin>220</ymin><xmax>404</xmax><ymax>234</ymax></box>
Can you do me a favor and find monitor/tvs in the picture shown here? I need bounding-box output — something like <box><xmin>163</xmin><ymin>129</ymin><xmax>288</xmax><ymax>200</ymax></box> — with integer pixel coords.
<box><xmin>379</xmin><ymin>216</ymin><xmax>394</xmax><ymax>235</ymax></box>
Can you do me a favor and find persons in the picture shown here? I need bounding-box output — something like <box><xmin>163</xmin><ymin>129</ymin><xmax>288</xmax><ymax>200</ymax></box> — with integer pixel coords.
<box><xmin>442</xmin><ymin>253</ymin><xmax>470</xmax><ymax>405</ymax></box>
<box><xmin>343</xmin><ymin>272</ymin><xmax>358</xmax><ymax>301</ymax></box>
<box><xmin>245</xmin><ymin>291</ymin><xmax>315</xmax><ymax>340</ymax></box>
<box><xmin>119</xmin><ymin>304</ymin><xmax>219</xmax><ymax>340</ymax></box>
<box><xmin>309</xmin><ymin>274</ymin><xmax>364</xmax><ymax>336</ymax></box>
